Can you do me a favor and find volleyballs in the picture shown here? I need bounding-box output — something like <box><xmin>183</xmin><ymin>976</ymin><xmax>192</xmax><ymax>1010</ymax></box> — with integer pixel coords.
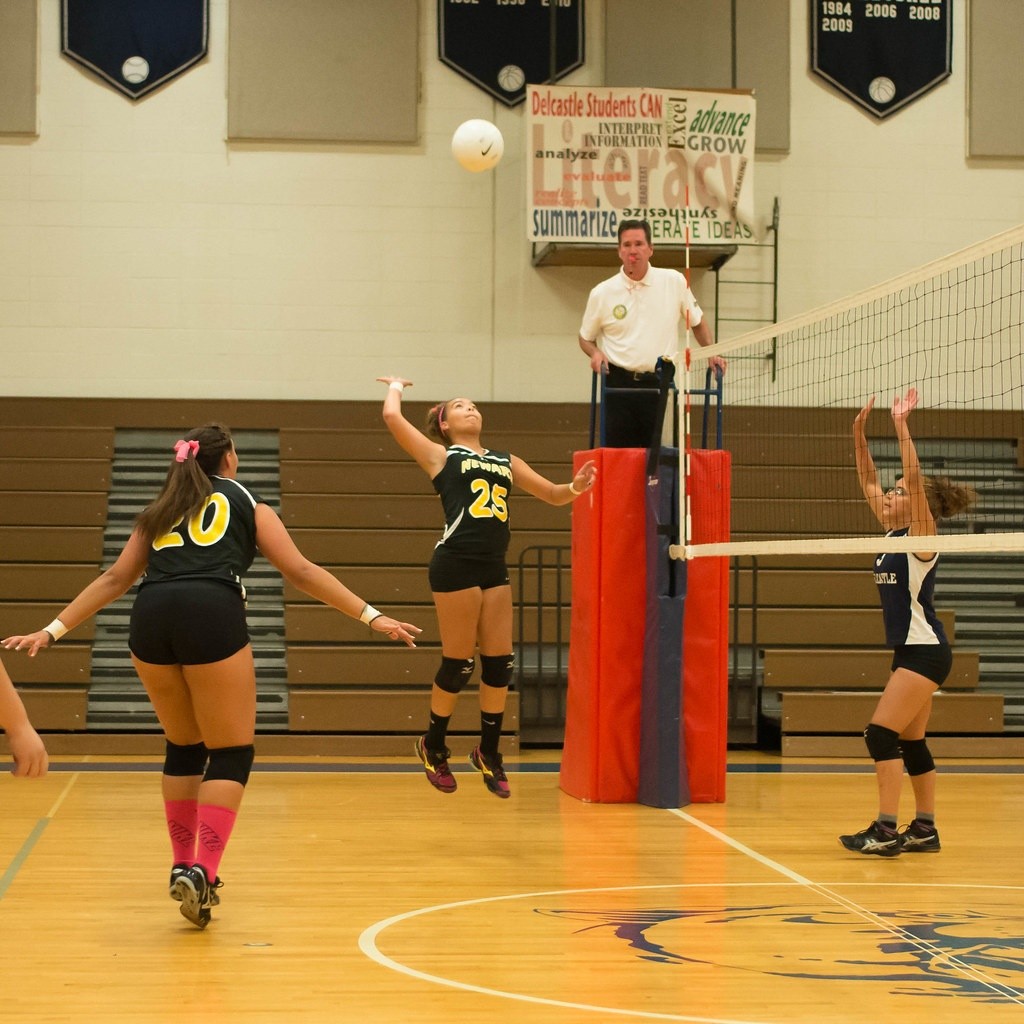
<box><xmin>451</xmin><ymin>119</ymin><xmax>505</xmax><ymax>172</ymax></box>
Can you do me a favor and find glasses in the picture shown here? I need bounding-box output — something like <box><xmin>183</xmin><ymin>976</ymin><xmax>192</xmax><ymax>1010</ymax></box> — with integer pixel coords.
<box><xmin>887</xmin><ymin>488</ymin><xmax>907</xmax><ymax>496</ymax></box>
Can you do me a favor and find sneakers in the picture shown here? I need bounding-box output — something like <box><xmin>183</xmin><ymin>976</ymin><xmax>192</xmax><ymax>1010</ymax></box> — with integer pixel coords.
<box><xmin>175</xmin><ymin>863</ymin><xmax>212</xmax><ymax>929</ymax></box>
<box><xmin>467</xmin><ymin>745</ymin><xmax>510</xmax><ymax>798</ymax></box>
<box><xmin>169</xmin><ymin>865</ymin><xmax>224</xmax><ymax>906</ymax></box>
<box><xmin>897</xmin><ymin>820</ymin><xmax>941</xmax><ymax>852</ymax></box>
<box><xmin>837</xmin><ymin>821</ymin><xmax>902</xmax><ymax>857</ymax></box>
<box><xmin>414</xmin><ymin>735</ymin><xmax>457</xmax><ymax>793</ymax></box>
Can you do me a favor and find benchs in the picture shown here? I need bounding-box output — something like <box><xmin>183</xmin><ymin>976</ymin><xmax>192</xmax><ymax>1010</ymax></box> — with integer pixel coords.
<box><xmin>0</xmin><ymin>395</ymin><xmax>1023</xmax><ymax>758</ymax></box>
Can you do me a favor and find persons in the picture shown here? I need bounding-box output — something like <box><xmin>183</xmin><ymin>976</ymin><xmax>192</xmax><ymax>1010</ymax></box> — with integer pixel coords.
<box><xmin>0</xmin><ymin>655</ymin><xmax>49</xmax><ymax>778</ymax></box>
<box><xmin>373</xmin><ymin>374</ymin><xmax>599</xmax><ymax>798</ymax></box>
<box><xmin>0</xmin><ymin>418</ymin><xmax>424</xmax><ymax>931</ymax></box>
<box><xmin>836</xmin><ymin>387</ymin><xmax>970</xmax><ymax>859</ymax></box>
<box><xmin>578</xmin><ymin>220</ymin><xmax>727</xmax><ymax>475</ymax></box>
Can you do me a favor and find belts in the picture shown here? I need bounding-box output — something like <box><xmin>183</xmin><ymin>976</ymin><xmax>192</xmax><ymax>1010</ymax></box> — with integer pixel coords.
<box><xmin>609</xmin><ymin>363</ymin><xmax>654</xmax><ymax>381</ymax></box>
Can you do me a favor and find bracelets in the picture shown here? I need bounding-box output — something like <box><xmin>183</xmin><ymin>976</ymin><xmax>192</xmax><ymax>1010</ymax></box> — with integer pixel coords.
<box><xmin>388</xmin><ymin>381</ymin><xmax>403</xmax><ymax>394</ymax></box>
<box><xmin>568</xmin><ymin>482</ymin><xmax>583</xmax><ymax>498</ymax></box>
<box><xmin>359</xmin><ymin>603</ymin><xmax>381</xmax><ymax>628</ymax></box>
<box><xmin>42</xmin><ymin>617</ymin><xmax>70</xmax><ymax>643</ymax></box>
